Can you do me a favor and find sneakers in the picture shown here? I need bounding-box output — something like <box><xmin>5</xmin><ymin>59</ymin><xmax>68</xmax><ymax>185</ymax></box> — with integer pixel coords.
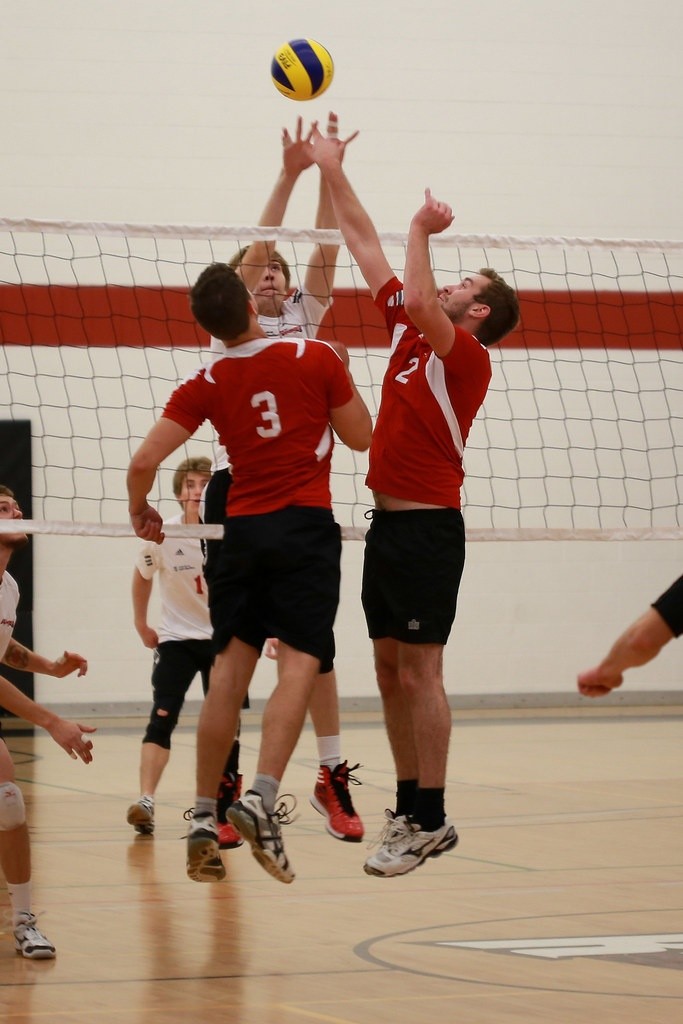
<box><xmin>3</xmin><ymin>904</ymin><xmax>56</xmax><ymax>960</ymax></box>
<box><xmin>216</xmin><ymin>773</ymin><xmax>245</xmax><ymax>848</ymax></box>
<box><xmin>228</xmin><ymin>788</ymin><xmax>300</xmax><ymax>882</ymax></box>
<box><xmin>182</xmin><ymin>808</ymin><xmax>226</xmax><ymax>882</ymax></box>
<box><xmin>127</xmin><ymin>794</ymin><xmax>157</xmax><ymax>835</ymax></box>
<box><xmin>364</xmin><ymin>811</ymin><xmax>460</xmax><ymax>875</ymax></box>
<box><xmin>312</xmin><ymin>758</ymin><xmax>363</xmax><ymax>843</ymax></box>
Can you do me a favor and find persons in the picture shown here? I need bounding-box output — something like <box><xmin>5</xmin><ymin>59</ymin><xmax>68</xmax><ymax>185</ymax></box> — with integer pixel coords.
<box><xmin>127</xmin><ymin>263</ymin><xmax>371</xmax><ymax>882</ymax></box>
<box><xmin>1</xmin><ymin>486</ymin><xmax>99</xmax><ymax>959</ymax></box>
<box><xmin>126</xmin><ymin>456</ymin><xmax>212</xmax><ymax>835</ymax></box>
<box><xmin>207</xmin><ymin>112</ymin><xmax>363</xmax><ymax>850</ymax></box>
<box><xmin>303</xmin><ymin>114</ymin><xmax>519</xmax><ymax>877</ymax></box>
<box><xmin>579</xmin><ymin>575</ymin><xmax>682</xmax><ymax>697</ymax></box>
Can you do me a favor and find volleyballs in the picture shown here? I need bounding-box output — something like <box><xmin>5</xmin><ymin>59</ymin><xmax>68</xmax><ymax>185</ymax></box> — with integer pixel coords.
<box><xmin>270</xmin><ymin>36</ymin><xmax>337</xmax><ymax>102</ymax></box>
